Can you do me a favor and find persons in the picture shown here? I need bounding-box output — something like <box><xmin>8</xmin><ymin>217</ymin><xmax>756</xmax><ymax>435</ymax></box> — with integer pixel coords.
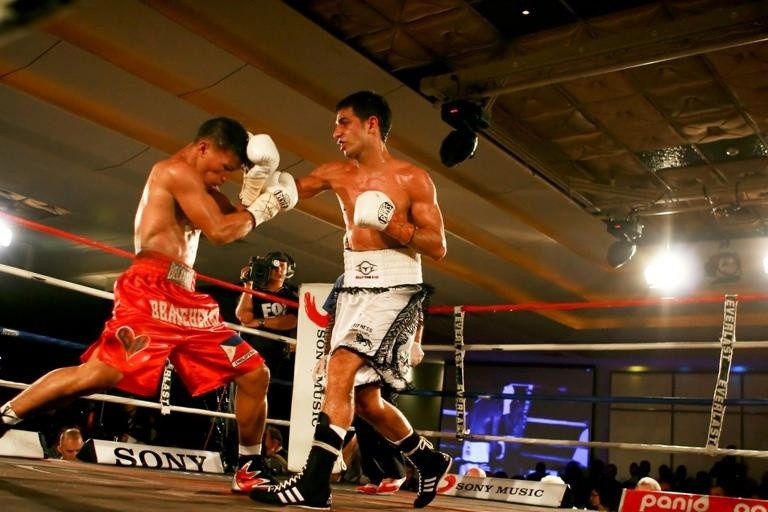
<box><xmin>249</xmin><ymin>90</ymin><xmax>453</xmax><ymax>510</ymax></box>
<box><xmin>235</xmin><ymin>251</ymin><xmax>299</xmax><ymax>451</ymax></box>
<box><xmin>37</xmin><ymin>394</ymin><xmax>153</xmax><ymax>459</ymax></box>
<box><xmin>0</xmin><ymin>117</ymin><xmax>299</xmax><ymax>494</ymax></box>
<box><xmin>440</xmin><ymin>384</ymin><xmax>527</xmax><ymax>475</ymax></box>
<box><xmin>264</xmin><ymin>426</ymin><xmax>288</xmax><ymax>473</ymax></box>
<box><xmin>312</xmin><ymin>233</ymin><xmax>425</xmax><ymax>496</ymax></box>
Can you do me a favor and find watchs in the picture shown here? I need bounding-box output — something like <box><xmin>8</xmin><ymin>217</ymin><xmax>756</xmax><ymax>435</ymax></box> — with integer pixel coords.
<box><xmin>258</xmin><ymin>318</ymin><xmax>266</xmax><ymax>327</ymax></box>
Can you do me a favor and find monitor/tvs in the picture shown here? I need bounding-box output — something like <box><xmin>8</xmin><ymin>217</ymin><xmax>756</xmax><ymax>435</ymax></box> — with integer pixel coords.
<box><xmin>436</xmin><ymin>358</ymin><xmax>597</xmax><ymax>485</ymax></box>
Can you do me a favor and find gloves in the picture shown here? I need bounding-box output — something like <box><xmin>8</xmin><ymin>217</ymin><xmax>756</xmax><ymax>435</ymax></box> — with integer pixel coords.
<box><xmin>237</xmin><ymin>134</ymin><xmax>283</xmax><ymax>208</ymax></box>
<box><xmin>351</xmin><ymin>189</ymin><xmax>416</xmax><ymax>246</ymax></box>
<box><xmin>244</xmin><ymin>167</ymin><xmax>301</xmax><ymax>229</ymax></box>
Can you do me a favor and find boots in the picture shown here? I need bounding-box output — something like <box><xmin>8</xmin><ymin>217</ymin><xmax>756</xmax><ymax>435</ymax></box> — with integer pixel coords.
<box><xmin>244</xmin><ymin>412</ymin><xmax>337</xmax><ymax>510</ymax></box>
<box><xmin>390</xmin><ymin>428</ymin><xmax>454</xmax><ymax>508</ymax></box>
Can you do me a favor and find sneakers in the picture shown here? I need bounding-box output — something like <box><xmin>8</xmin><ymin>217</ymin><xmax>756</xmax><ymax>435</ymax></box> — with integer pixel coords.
<box><xmin>230</xmin><ymin>453</ymin><xmax>285</xmax><ymax>496</ymax></box>
<box><xmin>354</xmin><ymin>475</ymin><xmax>407</xmax><ymax>495</ymax></box>
